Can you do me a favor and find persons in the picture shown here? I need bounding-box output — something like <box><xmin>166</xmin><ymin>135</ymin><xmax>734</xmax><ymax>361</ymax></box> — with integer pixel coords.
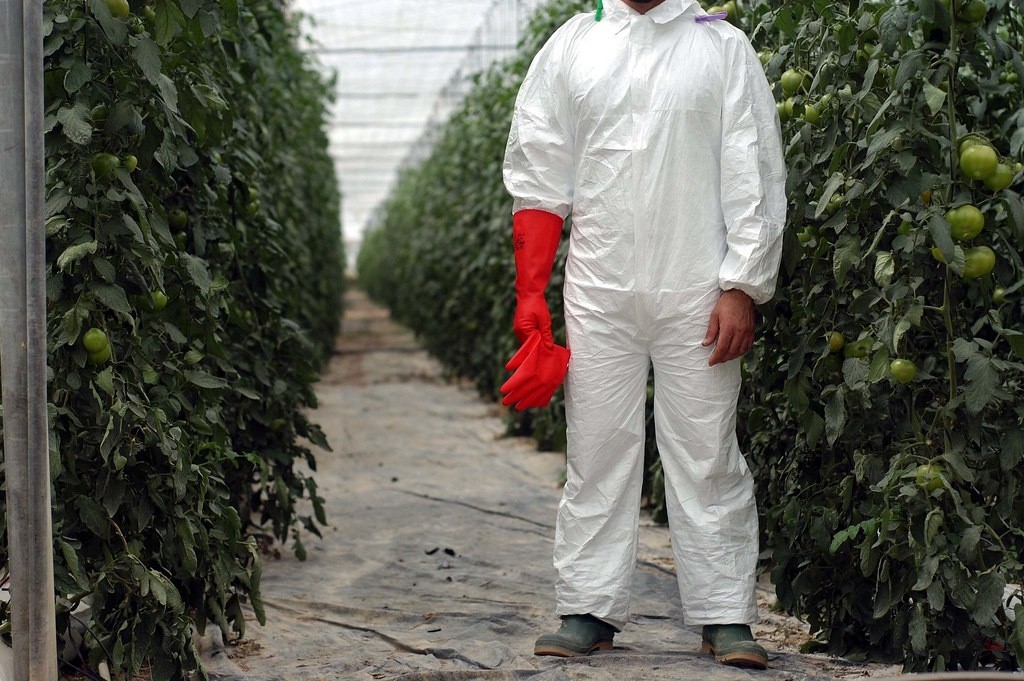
<box><xmin>500</xmin><ymin>1</ymin><xmax>786</xmax><ymax>670</ymax></box>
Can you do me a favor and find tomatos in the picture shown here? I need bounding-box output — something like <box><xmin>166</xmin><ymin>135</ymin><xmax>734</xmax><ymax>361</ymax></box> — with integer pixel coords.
<box><xmin>700</xmin><ymin>0</ymin><xmax>1013</xmax><ymax>489</ymax></box>
<box><xmin>81</xmin><ymin>0</ymin><xmax>262</xmax><ymax>370</ymax></box>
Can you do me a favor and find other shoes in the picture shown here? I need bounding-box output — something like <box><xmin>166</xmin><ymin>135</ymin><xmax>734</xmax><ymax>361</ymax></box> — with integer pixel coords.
<box><xmin>534</xmin><ymin>614</ymin><xmax>614</xmax><ymax>657</ymax></box>
<box><xmin>701</xmin><ymin>623</ymin><xmax>768</xmax><ymax>670</ymax></box>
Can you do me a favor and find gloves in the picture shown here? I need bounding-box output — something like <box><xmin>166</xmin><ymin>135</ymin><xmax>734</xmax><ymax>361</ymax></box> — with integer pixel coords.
<box><xmin>500</xmin><ymin>330</ymin><xmax>571</xmax><ymax>412</ymax></box>
<box><xmin>514</xmin><ymin>209</ymin><xmax>554</xmax><ymax>350</ymax></box>
